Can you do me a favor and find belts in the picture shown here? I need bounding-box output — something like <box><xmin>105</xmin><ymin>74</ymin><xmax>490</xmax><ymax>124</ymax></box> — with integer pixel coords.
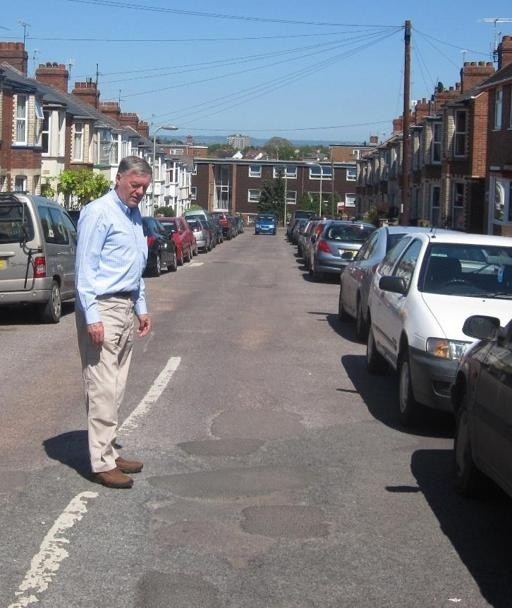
<box><xmin>114</xmin><ymin>290</ymin><xmax>133</xmax><ymax>299</ymax></box>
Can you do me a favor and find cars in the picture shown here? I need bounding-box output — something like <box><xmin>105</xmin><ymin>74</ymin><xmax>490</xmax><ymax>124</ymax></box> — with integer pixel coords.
<box><xmin>286</xmin><ymin>210</ymin><xmax>378</xmax><ymax>279</ymax></box>
<box><xmin>451</xmin><ymin>315</ymin><xmax>511</xmax><ymax>497</ymax></box>
<box><xmin>142</xmin><ymin>208</ymin><xmax>244</xmax><ymax>277</ymax></box>
<box><xmin>365</xmin><ymin>229</ymin><xmax>511</xmax><ymax>423</ymax></box>
<box><xmin>338</xmin><ymin>223</ymin><xmax>451</xmax><ymax>337</ymax></box>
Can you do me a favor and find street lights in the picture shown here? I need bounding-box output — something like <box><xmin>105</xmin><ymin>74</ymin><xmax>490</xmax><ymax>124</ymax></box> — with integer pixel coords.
<box><xmin>306</xmin><ymin>161</ymin><xmax>323</xmax><ymax>219</ymax></box>
<box><xmin>151</xmin><ymin>124</ymin><xmax>177</xmax><ymax>213</ymax></box>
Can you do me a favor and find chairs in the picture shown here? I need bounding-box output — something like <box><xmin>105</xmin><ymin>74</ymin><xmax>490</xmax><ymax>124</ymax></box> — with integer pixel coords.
<box><xmin>428</xmin><ymin>252</ymin><xmax>464</xmax><ymax>291</ymax></box>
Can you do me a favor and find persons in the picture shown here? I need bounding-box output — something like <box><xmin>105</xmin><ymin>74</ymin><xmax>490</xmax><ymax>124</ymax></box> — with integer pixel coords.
<box><xmin>73</xmin><ymin>156</ymin><xmax>152</xmax><ymax>489</ymax></box>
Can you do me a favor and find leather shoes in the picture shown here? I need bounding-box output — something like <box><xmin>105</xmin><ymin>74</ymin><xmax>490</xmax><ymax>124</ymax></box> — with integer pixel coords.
<box><xmin>89</xmin><ymin>466</ymin><xmax>134</xmax><ymax>490</ymax></box>
<box><xmin>114</xmin><ymin>457</ymin><xmax>144</xmax><ymax>474</ymax></box>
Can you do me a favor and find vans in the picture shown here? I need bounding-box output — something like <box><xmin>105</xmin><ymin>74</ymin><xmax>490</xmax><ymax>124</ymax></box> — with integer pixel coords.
<box><xmin>255</xmin><ymin>212</ymin><xmax>277</xmax><ymax>235</ymax></box>
<box><xmin>0</xmin><ymin>189</ymin><xmax>77</xmax><ymax>323</ymax></box>
